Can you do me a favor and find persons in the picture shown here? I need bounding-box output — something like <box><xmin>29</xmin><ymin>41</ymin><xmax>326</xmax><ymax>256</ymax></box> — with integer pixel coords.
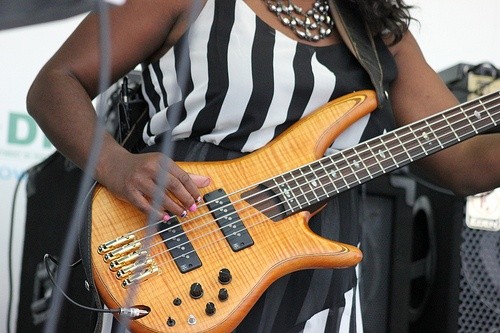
<box><xmin>26</xmin><ymin>0</ymin><xmax>500</xmax><ymax>333</ymax></box>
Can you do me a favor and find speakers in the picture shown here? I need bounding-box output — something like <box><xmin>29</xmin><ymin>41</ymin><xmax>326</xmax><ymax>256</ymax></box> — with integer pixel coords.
<box><xmin>14</xmin><ymin>140</ymin><xmax>115</xmax><ymax>333</ymax></box>
<box><xmin>354</xmin><ymin>168</ymin><xmax>500</xmax><ymax>333</ymax></box>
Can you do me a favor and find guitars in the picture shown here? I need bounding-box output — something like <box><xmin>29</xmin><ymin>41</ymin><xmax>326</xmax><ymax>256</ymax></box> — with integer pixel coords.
<box><xmin>77</xmin><ymin>89</ymin><xmax>500</xmax><ymax>333</ymax></box>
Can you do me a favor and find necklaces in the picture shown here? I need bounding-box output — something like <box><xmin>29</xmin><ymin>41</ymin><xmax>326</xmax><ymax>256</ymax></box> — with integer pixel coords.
<box><xmin>265</xmin><ymin>0</ymin><xmax>334</xmax><ymax>42</ymax></box>
